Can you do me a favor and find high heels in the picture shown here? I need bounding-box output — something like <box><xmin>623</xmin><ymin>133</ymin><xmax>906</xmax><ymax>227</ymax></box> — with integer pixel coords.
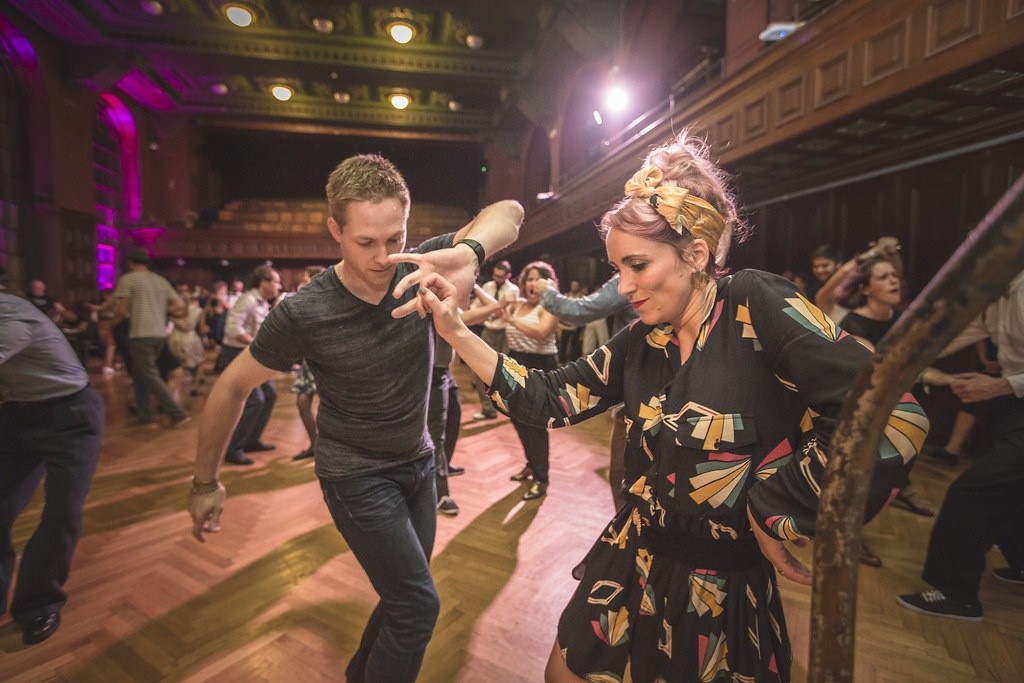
<box><xmin>510</xmin><ymin>464</ymin><xmax>532</xmax><ymax>477</ymax></box>
<box><xmin>522</xmin><ymin>481</ymin><xmax>547</xmax><ymax>500</ymax></box>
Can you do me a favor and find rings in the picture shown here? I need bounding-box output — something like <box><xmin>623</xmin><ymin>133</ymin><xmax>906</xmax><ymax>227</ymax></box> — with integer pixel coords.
<box><xmin>777</xmin><ymin>570</ymin><xmax>786</xmax><ymax>576</ymax></box>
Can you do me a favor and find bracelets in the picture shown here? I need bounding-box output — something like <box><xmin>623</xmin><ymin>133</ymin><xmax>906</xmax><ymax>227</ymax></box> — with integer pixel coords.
<box><xmin>191</xmin><ymin>478</ymin><xmax>222</xmax><ymax>494</ymax></box>
<box><xmin>451</xmin><ymin>238</ymin><xmax>485</xmax><ymax>267</ymax></box>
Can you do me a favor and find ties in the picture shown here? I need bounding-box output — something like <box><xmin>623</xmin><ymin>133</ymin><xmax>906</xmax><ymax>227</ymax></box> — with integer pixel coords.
<box><xmin>494</xmin><ymin>286</ymin><xmax>500</xmax><ymax>301</ymax></box>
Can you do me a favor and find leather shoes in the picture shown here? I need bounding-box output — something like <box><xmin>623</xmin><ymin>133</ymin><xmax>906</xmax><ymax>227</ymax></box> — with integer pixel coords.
<box><xmin>23</xmin><ymin>613</ymin><xmax>59</xmax><ymax>645</ymax></box>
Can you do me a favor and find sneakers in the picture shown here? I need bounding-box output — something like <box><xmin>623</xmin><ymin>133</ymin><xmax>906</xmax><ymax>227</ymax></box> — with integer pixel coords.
<box><xmin>896</xmin><ymin>588</ymin><xmax>984</xmax><ymax>622</ymax></box>
<box><xmin>436</xmin><ymin>495</ymin><xmax>459</xmax><ymax>515</ymax></box>
<box><xmin>448</xmin><ymin>464</ymin><xmax>465</xmax><ymax>476</ymax></box>
<box><xmin>991</xmin><ymin>565</ymin><xmax>1024</xmax><ymax>583</ymax></box>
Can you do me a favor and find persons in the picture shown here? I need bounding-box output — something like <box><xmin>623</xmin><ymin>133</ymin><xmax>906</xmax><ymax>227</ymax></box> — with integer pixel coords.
<box><xmin>418</xmin><ymin>129</ymin><xmax>930</xmax><ymax>683</ymax></box>
<box><xmin>780</xmin><ymin>238</ymin><xmax>1023</xmax><ymax>620</ymax></box>
<box><xmin>187</xmin><ymin>154</ymin><xmax>526</xmax><ymax>683</ymax></box>
<box><xmin>0</xmin><ymin>292</ymin><xmax>106</xmax><ymax>645</ymax></box>
<box><xmin>427</xmin><ymin>261</ymin><xmax>638</xmax><ymax>516</ymax></box>
<box><xmin>26</xmin><ymin>247</ymin><xmax>319</xmax><ymax>466</ymax></box>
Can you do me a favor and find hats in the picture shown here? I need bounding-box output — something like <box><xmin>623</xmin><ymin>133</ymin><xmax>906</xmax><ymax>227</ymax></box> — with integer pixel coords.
<box><xmin>130</xmin><ymin>248</ymin><xmax>148</xmax><ymax>261</ymax></box>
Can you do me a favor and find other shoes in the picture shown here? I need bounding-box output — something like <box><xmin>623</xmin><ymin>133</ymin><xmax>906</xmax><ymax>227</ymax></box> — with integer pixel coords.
<box><xmin>923</xmin><ymin>441</ymin><xmax>955</xmax><ymax>464</ymax></box>
<box><xmin>225</xmin><ymin>447</ymin><xmax>253</xmax><ymax>465</ymax></box>
<box><xmin>126</xmin><ymin>416</ymin><xmax>156</xmax><ymax>428</ymax></box>
<box><xmin>246</xmin><ymin>440</ymin><xmax>275</xmax><ymax>451</ymax></box>
<box><xmin>472</xmin><ymin>413</ymin><xmax>485</xmax><ymax>419</ymax></box>
<box><xmin>293</xmin><ymin>446</ymin><xmax>312</xmax><ymax>459</ymax></box>
<box><xmin>173</xmin><ymin>416</ymin><xmax>191</xmax><ymax>428</ymax></box>
<box><xmin>101</xmin><ymin>365</ymin><xmax>116</xmax><ymax>374</ymax></box>
